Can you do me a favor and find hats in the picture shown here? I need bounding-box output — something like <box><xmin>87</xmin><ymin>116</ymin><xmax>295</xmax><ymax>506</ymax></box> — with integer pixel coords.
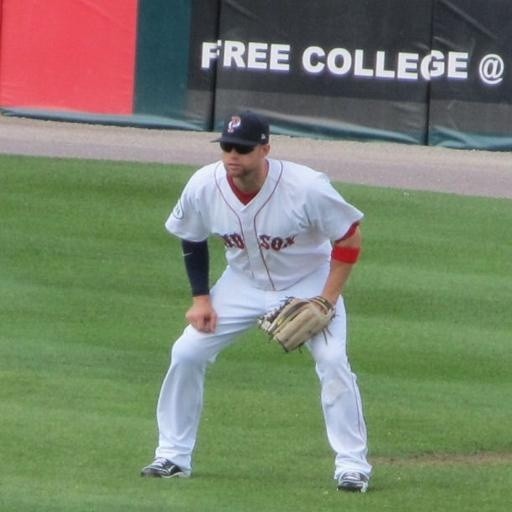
<box><xmin>210</xmin><ymin>110</ymin><xmax>270</xmax><ymax>146</ymax></box>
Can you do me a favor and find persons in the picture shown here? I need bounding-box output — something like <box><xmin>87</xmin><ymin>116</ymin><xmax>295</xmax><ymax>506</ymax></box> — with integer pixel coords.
<box><xmin>141</xmin><ymin>107</ymin><xmax>372</xmax><ymax>493</ymax></box>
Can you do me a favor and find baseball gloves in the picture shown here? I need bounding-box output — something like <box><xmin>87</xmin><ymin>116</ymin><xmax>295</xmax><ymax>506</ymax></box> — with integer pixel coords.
<box><xmin>259</xmin><ymin>296</ymin><xmax>334</xmax><ymax>354</ymax></box>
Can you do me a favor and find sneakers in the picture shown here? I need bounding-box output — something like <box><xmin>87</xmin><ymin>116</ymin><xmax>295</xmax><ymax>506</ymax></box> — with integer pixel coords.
<box><xmin>141</xmin><ymin>457</ymin><xmax>186</xmax><ymax>480</ymax></box>
<box><xmin>336</xmin><ymin>471</ymin><xmax>368</xmax><ymax>490</ymax></box>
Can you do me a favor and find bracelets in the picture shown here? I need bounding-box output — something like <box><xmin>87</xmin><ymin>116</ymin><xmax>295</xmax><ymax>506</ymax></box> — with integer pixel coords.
<box><xmin>333</xmin><ymin>243</ymin><xmax>361</xmax><ymax>262</ymax></box>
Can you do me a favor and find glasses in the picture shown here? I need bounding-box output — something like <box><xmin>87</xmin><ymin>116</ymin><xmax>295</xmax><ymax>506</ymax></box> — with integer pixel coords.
<box><xmin>220</xmin><ymin>142</ymin><xmax>255</xmax><ymax>154</ymax></box>
